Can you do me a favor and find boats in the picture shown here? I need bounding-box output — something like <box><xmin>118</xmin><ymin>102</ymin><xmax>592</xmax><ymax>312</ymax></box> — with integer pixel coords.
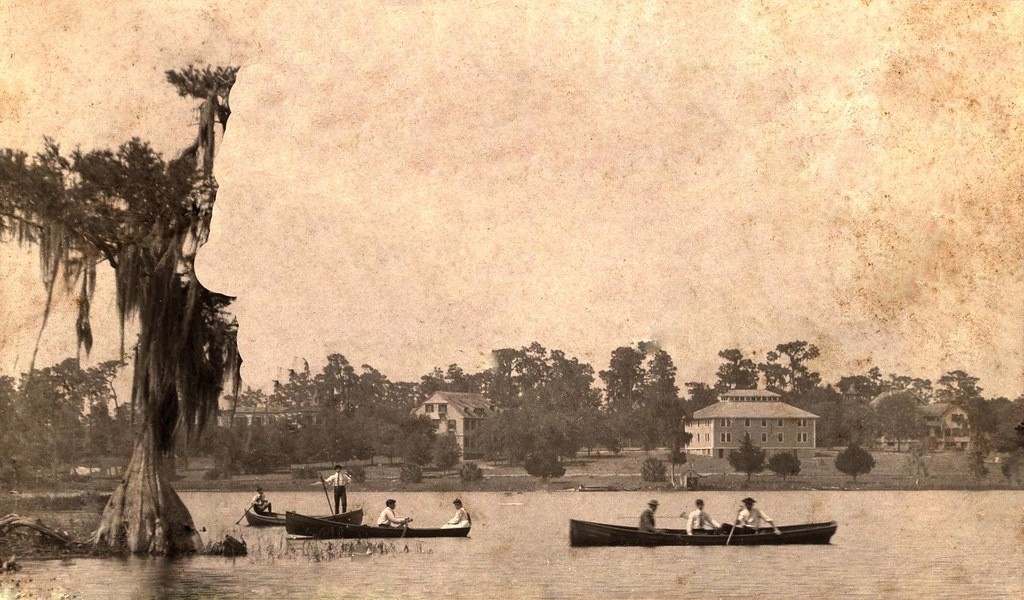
<box><xmin>569</xmin><ymin>519</ymin><xmax>838</xmax><ymax>547</ymax></box>
<box><xmin>286</xmin><ymin>511</ymin><xmax>471</xmax><ymax>539</ymax></box>
<box><xmin>244</xmin><ymin>508</ymin><xmax>364</xmax><ymax>526</ymax></box>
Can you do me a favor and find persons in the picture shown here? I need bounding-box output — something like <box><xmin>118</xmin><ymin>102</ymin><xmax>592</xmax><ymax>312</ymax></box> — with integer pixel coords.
<box><xmin>321</xmin><ymin>464</ymin><xmax>352</xmax><ymax>515</ymax></box>
<box><xmin>639</xmin><ymin>499</ymin><xmax>668</xmax><ymax>532</ymax></box>
<box><xmin>686</xmin><ymin>499</ymin><xmax>718</xmax><ymax>535</ymax></box>
<box><xmin>442</xmin><ymin>497</ymin><xmax>471</xmax><ymax>528</ymax></box>
<box><xmin>379</xmin><ymin>499</ymin><xmax>411</xmax><ymax>527</ymax></box>
<box><xmin>735</xmin><ymin>497</ymin><xmax>782</xmax><ymax>535</ymax></box>
<box><xmin>254</xmin><ymin>487</ymin><xmax>272</xmax><ymax>512</ymax></box>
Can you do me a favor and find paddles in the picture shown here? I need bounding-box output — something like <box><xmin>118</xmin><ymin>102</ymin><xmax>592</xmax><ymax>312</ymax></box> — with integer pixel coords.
<box><xmin>236</xmin><ymin>498</ymin><xmax>260</xmax><ymax>524</ymax></box>
<box><xmin>319</xmin><ymin>476</ymin><xmax>334</xmax><ymax>516</ymax></box>
<box><xmin>726</xmin><ymin>505</ymin><xmax>743</xmax><ymax>546</ymax></box>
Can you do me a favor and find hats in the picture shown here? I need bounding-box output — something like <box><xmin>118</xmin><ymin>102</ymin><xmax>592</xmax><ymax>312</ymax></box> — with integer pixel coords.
<box><xmin>647</xmin><ymin>500</ymin><xmax>660</xmax><ymax>505</ymax></box>
<box><xmin>741</xmin><ymin>497</ymin><xmax>755</xmax><ymax>504</ymax></box>
<box><xmin>334</xmin><ymin>465</ymin><xmax>342</xmax><ymax>471</ymax></box>
<box><xmin>386</xmin><ymin>499</ymin><xmax>396</xmax><ymax>503</ymax></box>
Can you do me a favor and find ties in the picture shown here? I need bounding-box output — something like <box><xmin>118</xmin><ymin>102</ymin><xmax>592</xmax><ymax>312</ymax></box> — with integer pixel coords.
<box><xmin>337</xmin><ymin>473</ymin><xmax>340</xmax><ymax>488</ymax></box>
<box><xmin>699</xmin><ymin>512</ymin><xmax>704</xmax><ymax>527</ymax></box>
<box><xmin>391</xmin><ymin>511</ymin><xmax>396</xmax><ymax>517</ymax></box>
